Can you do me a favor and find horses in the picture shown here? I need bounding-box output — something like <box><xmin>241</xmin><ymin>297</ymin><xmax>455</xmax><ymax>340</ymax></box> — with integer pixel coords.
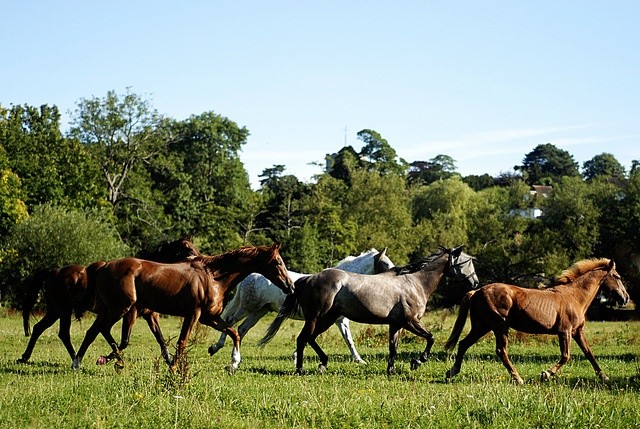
<box><xmin>19</xmin><ymin>233</ymin><xmax>202</xmax><ymax>368</ymax></box>
<box><xmin>258</xmin><ymin>243</ymin><xmax>480</xmax><ymax>376</ymax></box>
<box><xmin>71</xmin><ymin>240</ymin><xmax>295</xmax><ymax>378</ymax></box>
<box><xmin>444</xmin><ymin>257</ymin><xmax>631</xmax><ymax>385</ymax></box>
<box><xmin>207</xmin><ymin>245</ymin><xmax>397</xmax><ymax>377</ymax></box>
